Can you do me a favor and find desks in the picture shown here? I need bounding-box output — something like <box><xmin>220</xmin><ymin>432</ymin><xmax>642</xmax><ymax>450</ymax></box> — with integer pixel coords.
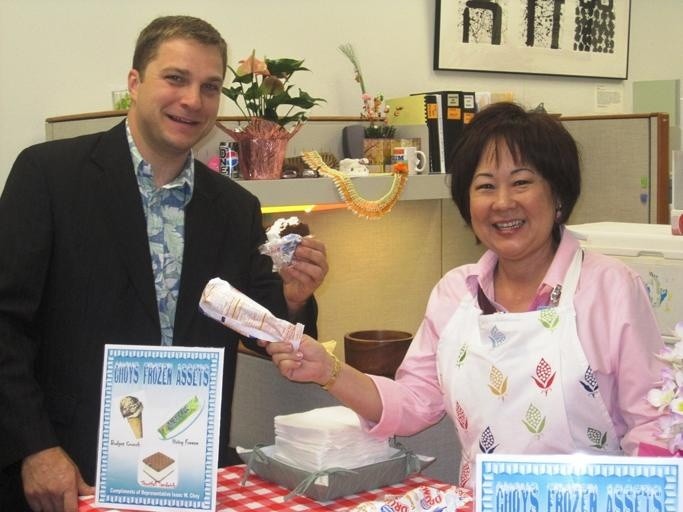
<box><xmin>78</xmin><ymin>458</ymin><xmax>473</xmax><ymax>512</ymax></box>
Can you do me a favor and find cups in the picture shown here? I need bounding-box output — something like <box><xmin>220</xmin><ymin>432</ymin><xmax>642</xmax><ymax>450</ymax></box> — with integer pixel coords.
<box><xmin>392</xmin><ymin>145</ymin><xmax>427</xmax><ymax>175</ymax></box>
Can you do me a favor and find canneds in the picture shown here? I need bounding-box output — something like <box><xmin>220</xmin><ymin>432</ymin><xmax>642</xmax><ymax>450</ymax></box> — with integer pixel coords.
<box><xmin>218</xmin><ymin>140</ymin><xmax>240</xmax><ymax>181</ymax></box>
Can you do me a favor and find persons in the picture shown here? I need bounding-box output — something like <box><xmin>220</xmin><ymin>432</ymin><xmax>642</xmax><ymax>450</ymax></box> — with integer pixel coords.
<box><xmin>0</xmin><ymin>15</ymin><xmax>328</xmax><ymax>511</ymax></box>
<box><xmin>257</xmin><ymin>99</ymin><xmax>683</xmax><ymax>492</ymax></box>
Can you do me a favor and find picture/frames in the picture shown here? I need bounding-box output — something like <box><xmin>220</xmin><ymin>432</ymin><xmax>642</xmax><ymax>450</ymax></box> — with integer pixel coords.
<box><xmin>435</xmin><ymin>0</ymin><xmax>630</xmax><ymax>80</ymax></box>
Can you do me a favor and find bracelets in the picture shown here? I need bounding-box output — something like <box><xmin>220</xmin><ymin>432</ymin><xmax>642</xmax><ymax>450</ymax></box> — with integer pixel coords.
<box><xmin>321</xmin><ymin>354</ymin><xmax>341</xmax><ymax>391</ymax></box>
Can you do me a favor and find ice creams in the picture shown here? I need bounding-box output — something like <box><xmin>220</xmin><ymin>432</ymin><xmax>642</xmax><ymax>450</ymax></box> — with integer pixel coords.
<box><xmin>119</xmin><ymin>396</ymin><xmax>144</xmax><ymax>439</ymax></box>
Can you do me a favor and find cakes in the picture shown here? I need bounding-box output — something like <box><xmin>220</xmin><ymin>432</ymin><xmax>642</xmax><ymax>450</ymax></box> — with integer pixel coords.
<box><xmin>142</xmin><ymin>452</ymin><xmax>176</xmax><ymax>482</ymax></box>
<box><xmin>265</xmin><ymin>216</ymin><xmax>309</xmax><ymax>243</ymax></box>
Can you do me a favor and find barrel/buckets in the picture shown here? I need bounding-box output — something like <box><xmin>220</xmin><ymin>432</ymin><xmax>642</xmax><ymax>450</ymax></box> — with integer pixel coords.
<box><xmin>344</xmin><ymin>329</ymin><xmax>412</xmax><ymax>380</ymax></box>
